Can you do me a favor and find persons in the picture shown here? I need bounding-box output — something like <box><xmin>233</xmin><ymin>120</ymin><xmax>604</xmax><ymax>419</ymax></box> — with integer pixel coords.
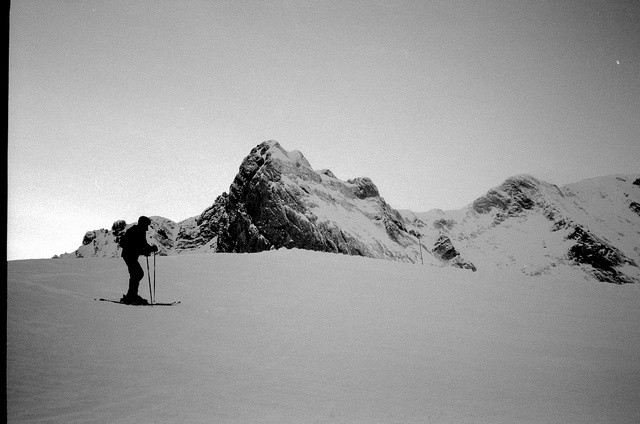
<box><xmin>119</xmin><ymin>215</ymin><xmax>158</xmax><ymax>303</ymax></box>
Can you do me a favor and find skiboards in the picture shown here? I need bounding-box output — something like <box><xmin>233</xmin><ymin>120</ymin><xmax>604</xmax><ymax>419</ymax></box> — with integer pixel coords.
<box><xmin>94</xmin><ymin>297</ymin><xmax>181</xmax><ymax>306</ymax></box>
